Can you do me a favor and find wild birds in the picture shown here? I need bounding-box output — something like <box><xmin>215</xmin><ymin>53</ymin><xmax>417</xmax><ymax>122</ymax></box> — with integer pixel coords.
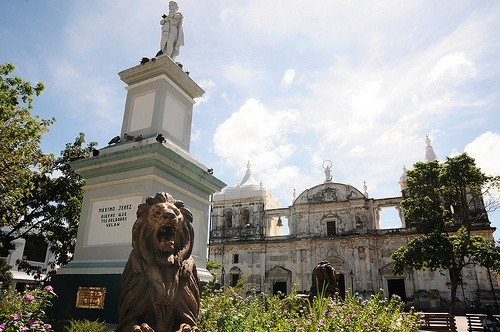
<box><xmin>68</xmin><ymin>153</ymin><xmax>85</xmax><ymax>162</ymax></box>
<box><xmin>124</xmin><ymin>133</ymin><xmax>142</xmax><ymax>142</ymax></box>
<box><xmin>140</xmin><ymin>57</ymin><xmax>156</xmax><ymax>65</ymax></box>
<box><xmin>92</xmin><ymin>149</ymin><xmax>100</xmax><ymax>157</ymax></box>
<box><xmin>162</xmin><ymin>14</ymin><xmax>167</xmax><ymax>19</ymax></box>
<box><xmin>156</xmin><ymin>133</ymin><xmax>165</xmax><ymax>143</ymax></box>
<box><xmin>175</xmin><ymin>62</ymin><xmax>190</xmax><ymax>75</ymax></box>
<box><xmin>108</xmin><ymin>136</ymin><xmax>120</xmax><ymax>144</ymax></box>
<box><xmin>155</xmin><ymin>49</ymin><xmax>163</xmax><ymax>57</ymax></box>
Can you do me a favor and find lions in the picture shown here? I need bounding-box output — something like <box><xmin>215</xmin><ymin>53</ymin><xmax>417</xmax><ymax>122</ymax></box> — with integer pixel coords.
<box><xmin>113</xmin><ymin>192</ymin><xmax>202</xmax><ymax>332</ymax></box>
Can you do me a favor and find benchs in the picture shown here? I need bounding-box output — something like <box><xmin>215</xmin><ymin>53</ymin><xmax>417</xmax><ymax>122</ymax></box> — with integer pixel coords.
<box><xmin>466</xmin><ymin>313</ymin><xmax>500</xmax><ymax>332</ymax></box>
<box><xmin>399</xmin><ymin>312</ymin><xmax>458</xmax><ymax>332</ymax></box>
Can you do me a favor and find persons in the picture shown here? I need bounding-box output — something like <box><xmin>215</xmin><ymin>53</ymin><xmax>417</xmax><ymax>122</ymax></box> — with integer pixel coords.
<box><xmin>247</xmin><ymin>159</ymin><xmax>251</xmax><ymax>171</ymax></box>
<box><xmin>324</xmin><ymin>165</ymin><xmax>332</xmax><ymax>180</ymax></box>
<box><xmin>292</xmin><ymin>188</ymin><xmax>297</xmax><ymax>199</ymax></box>
<box><xmin>402</xmin><ymin>164</ymin><xmax>406</xmax><ymax>172</ymax></box>
<box><xmin>424</xmin><ymin>134</ymin><xmax>431</xmax><ymax>145</ymax></box>
<box><xmin>159</xmin><ymin>0</ymin><xmax>185</xmax><ymax>60</ymax></box>
<box><xmin>363</xmin><ymin>182</ymin><xmax>367</xmax><ymax>191</ymax></box>
